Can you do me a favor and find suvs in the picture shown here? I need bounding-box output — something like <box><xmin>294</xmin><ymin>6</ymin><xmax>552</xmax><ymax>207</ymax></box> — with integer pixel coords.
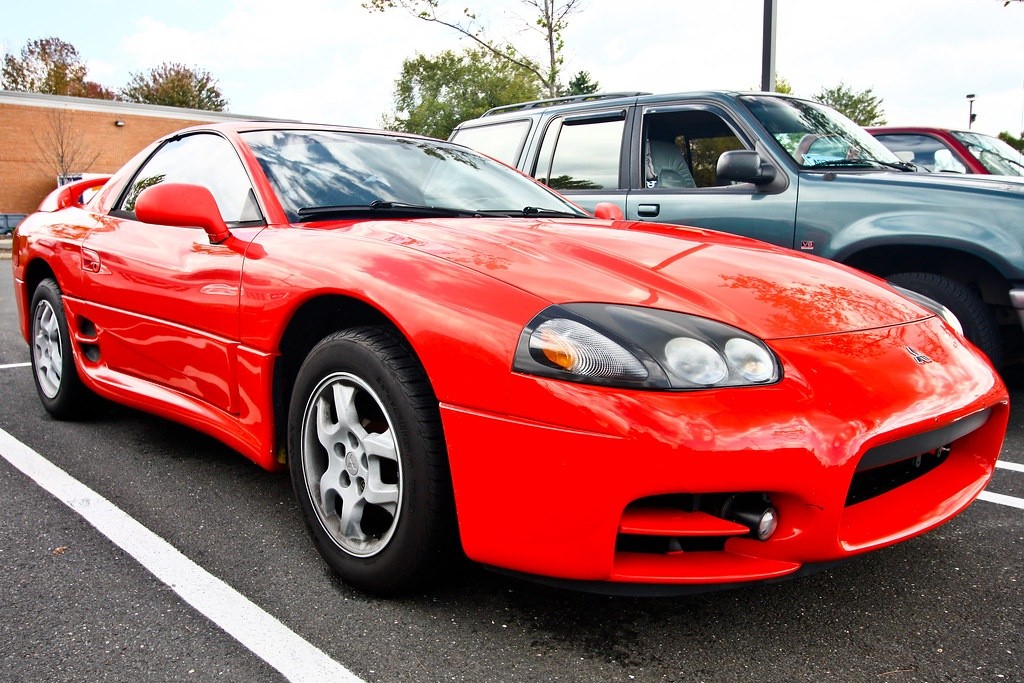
<box><xmin>448</xmin><ymin>91</ymin><xmax>1023</xmax><ymax>371</ymax></box>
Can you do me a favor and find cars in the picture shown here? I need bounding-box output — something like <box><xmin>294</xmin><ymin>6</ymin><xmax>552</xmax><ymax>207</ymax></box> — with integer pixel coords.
<box><xmin>863</xmin><ymin>127</ymin><xmax>1024</xmax><ymax>185</ymax></box>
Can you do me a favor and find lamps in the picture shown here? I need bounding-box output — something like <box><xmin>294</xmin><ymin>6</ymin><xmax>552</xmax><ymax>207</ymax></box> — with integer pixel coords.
<box><xmin>116</xmin><ymin>120</ymin><xmax>128</xmax><ymax>127</ymax></box>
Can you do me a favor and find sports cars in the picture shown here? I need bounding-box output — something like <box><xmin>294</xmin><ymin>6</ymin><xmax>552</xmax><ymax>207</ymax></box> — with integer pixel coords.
<box><xmin>11</xmin><ymin>122</ymin><xmax>1008</xmax><ymax>594</ymax></box>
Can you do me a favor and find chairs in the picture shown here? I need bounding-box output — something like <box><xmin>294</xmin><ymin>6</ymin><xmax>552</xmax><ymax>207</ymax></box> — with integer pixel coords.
<box><xmin>645</xmin><ymin>139</ymin><xmax>697</xmax><ymax>189</ymax></box>
